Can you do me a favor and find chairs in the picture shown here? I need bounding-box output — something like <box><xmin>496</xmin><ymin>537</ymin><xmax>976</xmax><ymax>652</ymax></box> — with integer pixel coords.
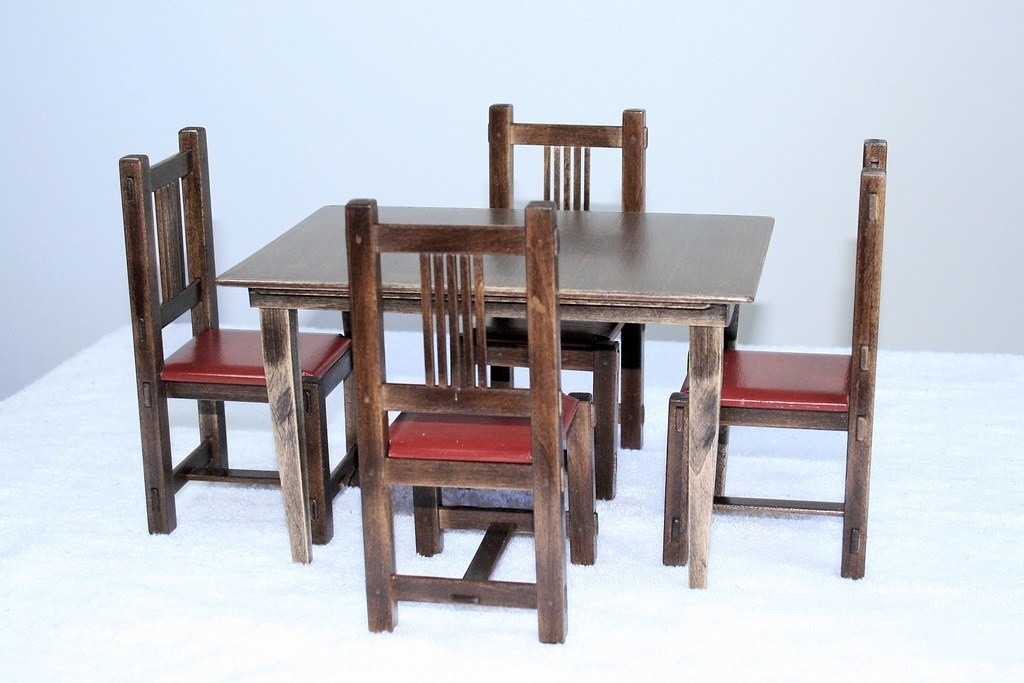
<box><xmin>664</xmin><ymin>140</ymin><xmax>888</xmax><ymax>578</ymax></box>
<box><xmin>345</xmin><ymin>197</ymin><xmax>597</xmax><ymax>645</ymax></box>
<box><xmin>449</xmin><ymin>103</ymin><xmax>647</xmax><ymax>502</ymax></box>
<box><xmin>119</xmin><ymin>127</ymin><xmax>356</xmax><ymax>547</ymax></box>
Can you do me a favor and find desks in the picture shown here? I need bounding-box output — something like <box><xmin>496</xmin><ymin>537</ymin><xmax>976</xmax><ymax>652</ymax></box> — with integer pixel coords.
<box><xmin>213</xmin><ymin>205</ymin><xmax>774</xmax><ymax>591</ymax></box>
